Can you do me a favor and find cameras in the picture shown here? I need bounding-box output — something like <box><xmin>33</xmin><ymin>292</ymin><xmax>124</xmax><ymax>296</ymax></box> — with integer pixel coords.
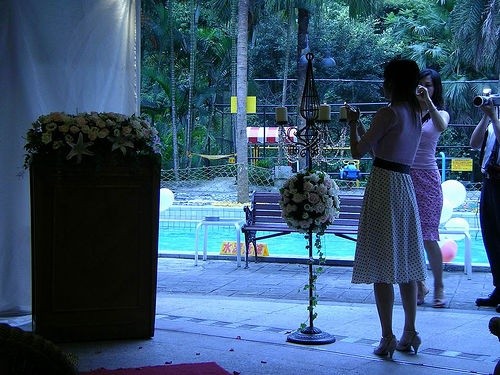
<box><xmin>472</xmin><ymin>94</ymin><xmax>500</xmax><ymax>108</ymax></box>
<box><xmin>416</xmin><ymin>87</ymin><xmax>423</xmax><ymax>95</ymax></box>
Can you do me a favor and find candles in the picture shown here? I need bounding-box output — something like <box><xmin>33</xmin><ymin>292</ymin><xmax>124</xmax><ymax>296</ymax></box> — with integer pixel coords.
<box><xmin>275</xmin><ymin>106</ymin><xmax>288</xmax><ymax>122</ymax></box>
<box><xmin>339</xmin><ymin>102</ymin><xmax>349</xmax><ymax>118</ymax></box>
<box><xmin>319</xmin><ymin>103</ymin><xmax>331</xmax><ymax>120</ymax></box>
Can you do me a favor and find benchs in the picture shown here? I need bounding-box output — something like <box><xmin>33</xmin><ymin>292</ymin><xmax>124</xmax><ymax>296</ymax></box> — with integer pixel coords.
<box><xmin>242</xmin><ymin>190</ymin><xmax>366</xmax><ymax>269</ymax></box>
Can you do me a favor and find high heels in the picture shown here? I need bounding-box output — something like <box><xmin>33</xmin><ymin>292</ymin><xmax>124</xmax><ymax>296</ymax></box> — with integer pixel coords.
<box><xmin>373</xmin><ymin>335</ymin><xmax>397</xmax><ymax>360</ymax></box>
<box><xmin>393</xmin><ymin>330</ymin><xmax>421</xmax><ymax>355</ymax></box>
<box><xmin>432</xmin><ymin>285</ymin><xmax>446</xmax><ymax>308</ymax></box>
<box><xmin>417</xmin><ymin>287</ymin><xmax>429</xmax><ymax>305</ymax></box>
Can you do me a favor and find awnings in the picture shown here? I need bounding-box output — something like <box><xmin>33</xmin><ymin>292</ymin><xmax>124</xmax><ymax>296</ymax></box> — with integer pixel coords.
<box><xmin>246</xmin><ymin>126</ymin><xmax>297</xmax><ymax>145</ymax></box>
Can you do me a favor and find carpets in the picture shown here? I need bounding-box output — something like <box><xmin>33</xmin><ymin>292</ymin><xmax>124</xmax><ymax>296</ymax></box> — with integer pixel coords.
<box><xmin>81</xmin><ymin>362</ymin><xmax>229</xmax><ymax>375</ymax></box>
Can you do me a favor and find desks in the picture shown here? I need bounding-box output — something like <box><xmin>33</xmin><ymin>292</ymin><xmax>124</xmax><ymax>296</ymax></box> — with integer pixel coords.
<box><xmin>435</xmin><ymin>218</ymin><xmax>473</xmax><ymax>280</ymax></box>
<box><xmin>194</xmin><ymin>219</ymin><xmax>248</xmax><ymax>267</ymax></box>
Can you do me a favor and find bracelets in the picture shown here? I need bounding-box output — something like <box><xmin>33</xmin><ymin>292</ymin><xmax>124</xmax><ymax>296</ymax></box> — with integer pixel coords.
<box><xmin>348</xmin><ymin>119</ymin><xmax>357</xmax><ymax>126</ymax></box>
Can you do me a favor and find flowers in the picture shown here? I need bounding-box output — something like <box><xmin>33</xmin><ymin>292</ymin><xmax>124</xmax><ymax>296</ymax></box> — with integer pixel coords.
<box><xmin>280</xmin><ymin>168</ymin><xmax>341</xmax><ymax>337</ymax></box>
<box><xmin>13</xmin><ymin>109</ymin><xmax>167</xmax><ymax>177</ymax></box>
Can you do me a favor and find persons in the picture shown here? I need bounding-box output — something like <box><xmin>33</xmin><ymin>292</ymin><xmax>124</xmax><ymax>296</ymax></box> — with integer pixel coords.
<box><xmin>409</xmin><ymin>69</ymin><xmax>451</xmax><ymax>308</ymax></box>
<box><xmin>469</xmin><ymin>99</ymin><xmax>500</xmax><ymax>313</ymax></box>
<box><xmin>345</xmin><ymin>57</ymin><xmax>428</xmax><ymax>358</ymax></box>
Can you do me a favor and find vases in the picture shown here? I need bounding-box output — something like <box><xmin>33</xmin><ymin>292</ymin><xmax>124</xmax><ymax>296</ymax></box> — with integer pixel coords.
<box><xmin>27</xmin><ymin>156</ymin><xmax>162</xmax><ymax>341</ymax></box>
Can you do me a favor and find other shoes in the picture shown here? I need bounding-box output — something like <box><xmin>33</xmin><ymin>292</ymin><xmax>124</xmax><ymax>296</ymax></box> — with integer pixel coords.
<box><xmin>475</xmin><ymin>288</ymin><xmax>500</xmax><ymax>307</ymax></box>
